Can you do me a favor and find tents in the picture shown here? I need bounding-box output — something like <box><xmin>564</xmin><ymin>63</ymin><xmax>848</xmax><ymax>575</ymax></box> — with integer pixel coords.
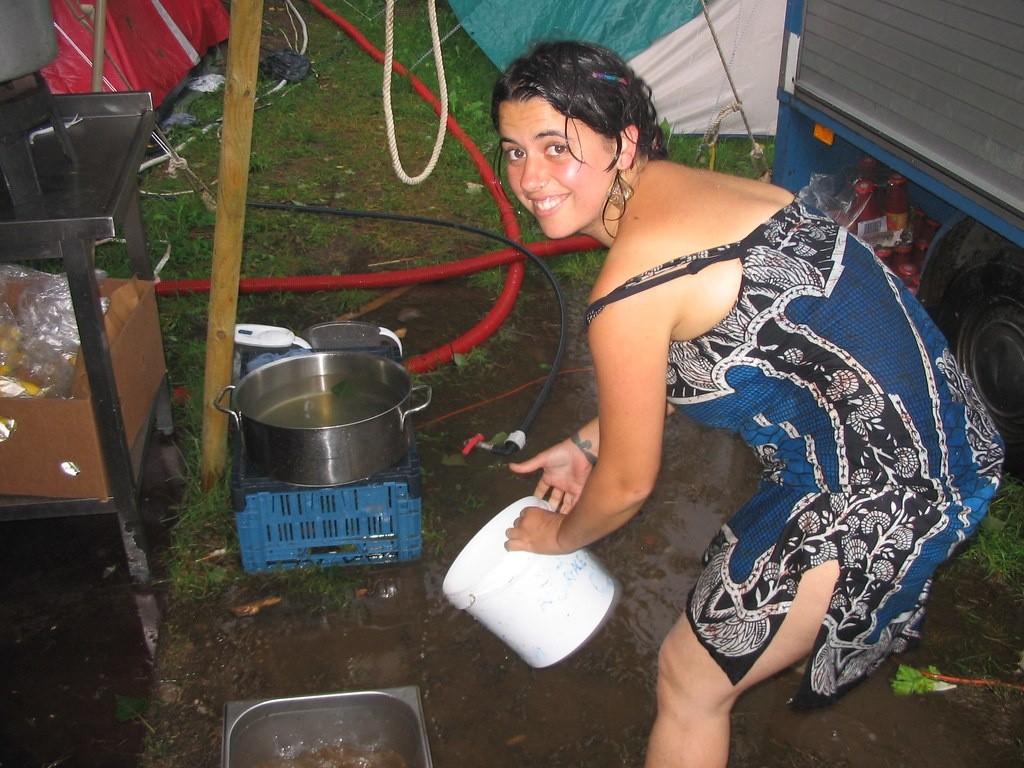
<box><xmin>446</xmin><ymin>0</ymin><xmax>787</xmax><ymax>138</ymax></box>
<box><xmin>37</xmin><ymin>0</ymin><xmax>231</xmax><ymax>125</ymax></box>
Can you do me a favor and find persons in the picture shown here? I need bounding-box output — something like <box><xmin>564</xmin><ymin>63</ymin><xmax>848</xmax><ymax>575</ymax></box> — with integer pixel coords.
<box><xmin>490</xmin><ymin>39</ymin><xmax>1005</xmax><ymax>768</ymax></box>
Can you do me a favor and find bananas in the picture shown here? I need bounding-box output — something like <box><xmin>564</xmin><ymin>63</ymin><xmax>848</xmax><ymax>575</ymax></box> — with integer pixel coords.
<box><xmin>0</xmin><ymin>323</ymin><xmax>45</xmax><ymax>397</ymax></box>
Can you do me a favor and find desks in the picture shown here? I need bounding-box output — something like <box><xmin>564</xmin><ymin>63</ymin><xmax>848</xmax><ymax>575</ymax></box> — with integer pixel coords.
<box><xmin>0</xmin><ymin>89</ymin><xmax>176</xmax><ymax>659</ymax></box>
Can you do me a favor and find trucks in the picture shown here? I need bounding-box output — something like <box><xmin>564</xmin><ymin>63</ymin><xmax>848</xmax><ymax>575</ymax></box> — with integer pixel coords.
<box><xmin>769</xmin><ymin>1</ymin><xmax>1024</xmax><ymax>483</ymax></box>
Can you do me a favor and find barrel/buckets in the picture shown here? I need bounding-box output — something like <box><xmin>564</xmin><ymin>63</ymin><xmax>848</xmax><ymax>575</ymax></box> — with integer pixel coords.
<box><xmin>441</xmin><ymin>494</ymin><xmax>615</xmax><ymax>669</ymax></box>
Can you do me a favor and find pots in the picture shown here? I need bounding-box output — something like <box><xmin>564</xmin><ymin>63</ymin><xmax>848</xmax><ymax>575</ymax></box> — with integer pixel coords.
<box><xmin>213</xmin><ymin>350</ymin><xmax>433</xmax><ymax>486</ymax></box>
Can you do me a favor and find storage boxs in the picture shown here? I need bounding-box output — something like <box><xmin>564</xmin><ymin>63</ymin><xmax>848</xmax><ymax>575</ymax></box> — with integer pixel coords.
<box><xmin>0</xmin><ymin>273</ymin><xmax>169</xmax><ymax>504</ymax></box>
<box><xmin>229</xmin><ymin>344</ymin><xmax>420</xmax><ymax>576</ymax></box>
